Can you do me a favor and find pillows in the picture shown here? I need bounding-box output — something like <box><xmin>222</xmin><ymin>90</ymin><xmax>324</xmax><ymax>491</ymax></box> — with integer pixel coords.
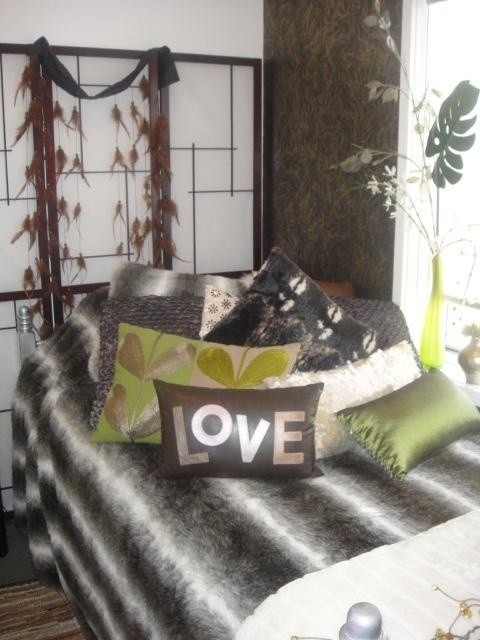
<box><xmin>333</xmin><ymin>364</ymin><xmax>478</xmax><ymax>481</ymax></box>
<box><xmin>151</xmin><ymin>376</ymin><xmax>324</xmax><ymax>481</ymax></box>
<box><xmin>91</xmin><ymin>319</ymin><xmax>300</xmax><ymax>446</ymax></box>
<box><xmin>88</xmin><ymin>294</ymin><xmax>204</xmax><ymax>431</ymax></box>
<box><xmin>263</xmin><ymin>342</ymin><xmax>424</xmax><ymax>459</ymax></box>
<box><xmin>201</xmin><ymin>248</ymin><xmax>418</xmax><ymax>361</ymax></box>
<box><xmin>109</xmin><ymin>260</ymin><xmax>206</xmax><ymax>296</ymax></box>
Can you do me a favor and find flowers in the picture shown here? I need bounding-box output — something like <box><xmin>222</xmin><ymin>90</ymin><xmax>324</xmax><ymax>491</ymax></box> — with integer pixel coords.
<box><xmin>328</xmin><ymin>1</ymin><xmax>480</xmax><ymax>254</ymax></box>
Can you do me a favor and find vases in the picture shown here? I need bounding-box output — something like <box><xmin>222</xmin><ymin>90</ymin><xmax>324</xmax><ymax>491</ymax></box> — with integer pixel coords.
<box><xmin>419</xmin><ymin>257</ymin><xmax>446</xmax><ymax>367</ymax></box>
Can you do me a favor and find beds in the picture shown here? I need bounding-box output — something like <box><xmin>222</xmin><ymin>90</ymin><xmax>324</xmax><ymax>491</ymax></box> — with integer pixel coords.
<box><xmin>8</xmin><ymin>284</ymin><xmax>479</xmax><ymax>640</ymax></box>
